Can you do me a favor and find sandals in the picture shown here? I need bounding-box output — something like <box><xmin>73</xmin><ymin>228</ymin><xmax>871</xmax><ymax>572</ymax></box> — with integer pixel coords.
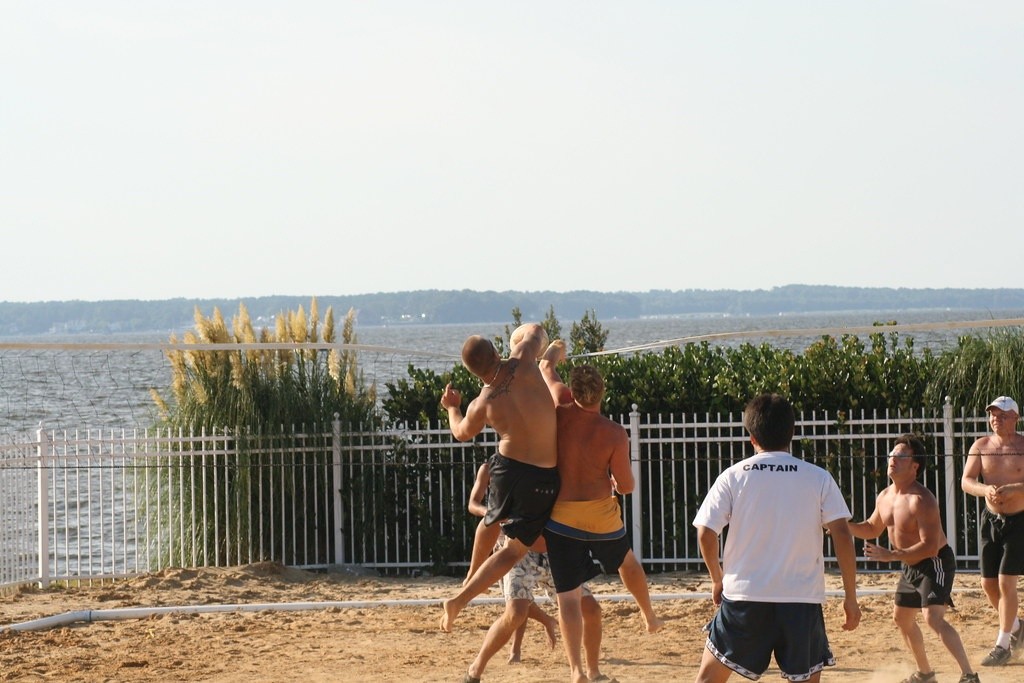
<box><xmin>959</xmin><ymin>672</ymin><xmax>980</xmax><ymax>683</ymax></box>
<box><xmin>900</xmin><ymin>670</ymin><xmax>938</xmax><ymax>683</ymax></box>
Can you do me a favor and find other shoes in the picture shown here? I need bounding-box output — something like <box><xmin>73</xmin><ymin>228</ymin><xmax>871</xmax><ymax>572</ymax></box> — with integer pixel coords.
<box><xmin>464</xmin><ymin>668</ymin><xmax>480</xmax><ymax>683</ymax></box>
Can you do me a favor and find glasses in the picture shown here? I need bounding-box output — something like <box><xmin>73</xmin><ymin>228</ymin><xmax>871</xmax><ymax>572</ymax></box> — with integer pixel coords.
<box><xmin>889</xmin><ymin>451</ymin><xmax>915</xmax><ymax>462</ymax></box>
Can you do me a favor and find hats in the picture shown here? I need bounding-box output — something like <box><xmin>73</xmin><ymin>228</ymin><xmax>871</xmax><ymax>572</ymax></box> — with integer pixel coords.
<box><xmin>985</xmin><ymin>395</ymin><xmax>1019</xmax><ymax>414</ymax></box>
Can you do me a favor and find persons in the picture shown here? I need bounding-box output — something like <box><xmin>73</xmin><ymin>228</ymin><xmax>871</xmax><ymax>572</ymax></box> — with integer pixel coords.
<box><xmin>692</xmin><ymin>395</ymin><xmax>862</xmax><ymax>683</ymax></box>
<box><xmin>825</xmin><ymin>434</ymin><xmax>982</xmax><ymax>683</ymax></box>
<box><xmin>960</xmin><ymin>395</ymin><xmax>1024</xmax><ymax>667</ymax></box>
<box><xmin>442</xmin><ymin>322</ymin><xmax>662</xmax><ymax>683</ymax></box>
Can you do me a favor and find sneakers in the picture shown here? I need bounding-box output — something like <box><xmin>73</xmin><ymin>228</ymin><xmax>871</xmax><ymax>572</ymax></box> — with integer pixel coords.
<box><xmin>1009</xmin><ymin>620</ymin><xmax>1024</xmax><ymax>660</ymax></box>
<box><xmin>980</xmin><ymin>644</ymin><xmax>1012</xmax><ymax>666</ymax></box>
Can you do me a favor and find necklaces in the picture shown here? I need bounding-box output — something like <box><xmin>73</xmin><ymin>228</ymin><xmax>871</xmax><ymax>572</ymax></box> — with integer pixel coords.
<box><xmin>478</xmin><ymin>363</ymin><xmax>501</xmax><ymax>389</ymax></box>
